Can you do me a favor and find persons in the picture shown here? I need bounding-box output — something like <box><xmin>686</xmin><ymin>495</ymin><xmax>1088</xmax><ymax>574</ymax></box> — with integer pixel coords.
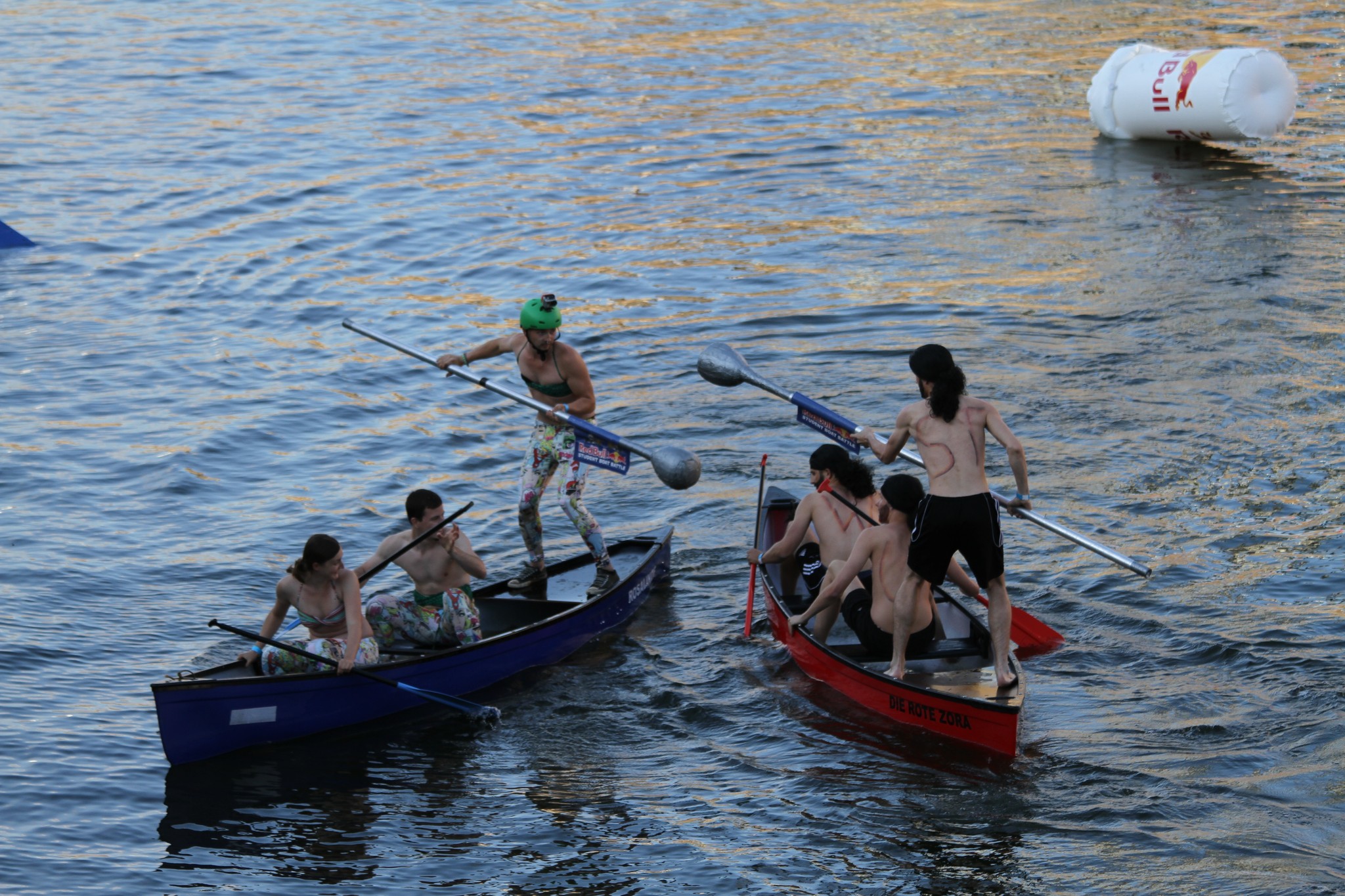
<box><xmin>239</xmin><ymin>533</ymin><xmax>377</xmax><ymax>671</ymax></box>
<box><xmin>746</xmin><ymin>444</ymin><xmax>879</xmax><ymax>595</ymax></box>
<box><xmin>353</xmin><ymin>487</ymin><xmax>487</xmax><ymax>658</ymax></box>
<box><xmin>848</xmin><ymin>345</ymin><xmax>1031</xmax><ymax>688</ymax></box>
<box><xmin>788</xmin><ymin>475</ymin><xmax>977</xmax><ymax>652</ymax></box>
<box><xmin>437</xmin><ymin>295</ymin><xmax>622</xmax><ymax>595</ymax></box>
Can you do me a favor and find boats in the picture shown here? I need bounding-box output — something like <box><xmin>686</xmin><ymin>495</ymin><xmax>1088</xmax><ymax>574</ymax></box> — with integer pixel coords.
<box><xmin>755</xmin><ymin>481</ymin><xmax>1026</xmax><ymax>760</ymax></box>
<box><xmin>148</xmin><ymin>521</ymin><xmax>676</xmax><ymax>767</ymax></box>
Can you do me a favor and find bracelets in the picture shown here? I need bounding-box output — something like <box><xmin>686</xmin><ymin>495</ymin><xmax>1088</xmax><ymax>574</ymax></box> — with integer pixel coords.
<box><xmin>563</xmin><ymin>403</ymin><xmax>569</xmax><ymax>412</ymax></box>
<box><xmin>251</xmin><ymin>645</ymin><xmax>261</xmax><ymax>654</ymax></box>
<box><xmin>758</xmin><ymin>553</ymin><xmax>764</xmax><ymax>564</ymax></box>
<box><xmin>463</xmin><ymin>352</ymin><xmax>470</xmax><ymax>367</ymax></box>
<box><xmin>1014</xmin><ymin>492</ymin><xmax>1030</xmax><ymax>500</ymax></box>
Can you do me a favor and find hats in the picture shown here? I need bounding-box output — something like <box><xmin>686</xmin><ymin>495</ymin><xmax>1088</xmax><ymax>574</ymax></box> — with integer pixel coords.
<box><xmin>810</xmin><ymin>444</ymin><xmax>848</xmax><ymax>470</ymax></box>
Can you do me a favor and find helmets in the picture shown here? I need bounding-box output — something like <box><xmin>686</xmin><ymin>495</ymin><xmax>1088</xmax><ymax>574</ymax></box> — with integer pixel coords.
<box><xmin>520</xmin><ymin>297</ymin><xmax>562</xmax><ymax>331</ymax></box>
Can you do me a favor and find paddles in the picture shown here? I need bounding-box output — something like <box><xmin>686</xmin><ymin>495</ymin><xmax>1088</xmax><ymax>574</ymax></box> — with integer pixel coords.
<box><xmin>273</xmin><ymin>501</ymin><xmax>476</xmax><ymax>639</ymax></box>
<box><xmin>817</xmin><ymin>477</ymin><xmax>1065</xmax><ymax>649</ymax></box>
<box><xmin>744</xmin><ymin>454</ymin><xmax>768</xmax><ymax>637</ymax></box>
<box><xmin>208</xmin><ymin>617</ymin><xmax>483</xmax><ymax>713</ymax></box>
